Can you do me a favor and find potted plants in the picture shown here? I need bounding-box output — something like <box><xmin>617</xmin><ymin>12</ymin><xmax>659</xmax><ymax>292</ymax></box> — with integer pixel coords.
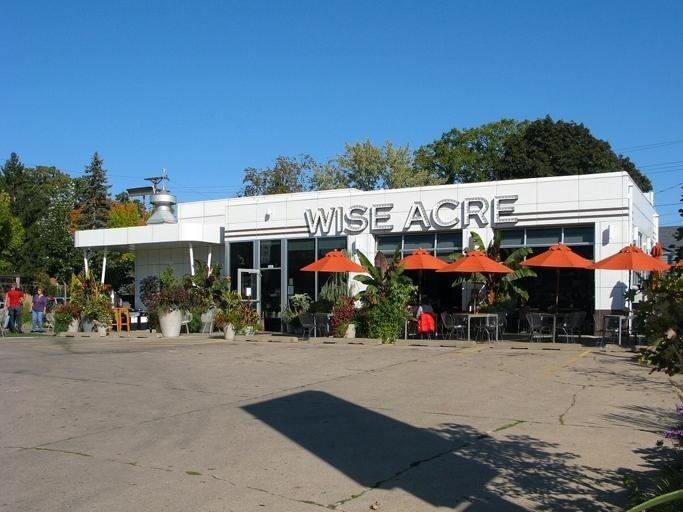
<box><xmin>140</xmin><ymin>261</ymin><xmax>262</xmax><ymax>340</ymax></box>
<box><xmin>52</xmin><ymin>271</ymin><xmax>113</xmax><ymax>337</ymax></box>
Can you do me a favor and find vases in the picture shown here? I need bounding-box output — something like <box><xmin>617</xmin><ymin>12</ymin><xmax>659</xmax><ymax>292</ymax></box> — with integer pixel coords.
<box><xmin>344</xmin><ymin>324</ymin><xmax>356</xmax><ymax>338</ymax></box>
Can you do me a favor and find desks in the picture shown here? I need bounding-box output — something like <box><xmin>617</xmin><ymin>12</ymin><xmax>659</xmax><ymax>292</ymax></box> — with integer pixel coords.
<box><xmin>603</xmin><ymin>314</ymin><xmax>629</xmax><ymax>345</ymax></box>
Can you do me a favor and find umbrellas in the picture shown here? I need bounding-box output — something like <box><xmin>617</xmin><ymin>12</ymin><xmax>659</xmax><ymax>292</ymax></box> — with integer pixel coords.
<box><xmin>435</xmin><ymin>250</ymin><xmax>515</xmax><ymax>314</ymax></box>
<box><xmin>397</xmin><ymin>248</ymin><xmax>450</xmax><ymax>304</ymax></box>
<box><xmin>668</xmin><ymin>260</ymin><xmax>683</xmax><ymax>267</ymax></box>
<box><xmin>651</xmin><ymin>246</ymin><xmax>663</xmax><ymax>289</ymax></box>
<box><xmin>586</xmin><ymin>243</ymin><xmax>671</xmax><ymax>335</ymax></box>
<box><xmin>300</xmin><ymin>249</ymin><xmax>367</xmax><ymax>300</ymax></box>
<box><xmin>518</xmin><ymin>242</ymin><xmax>595</xmax><ymax>315</ymax></box>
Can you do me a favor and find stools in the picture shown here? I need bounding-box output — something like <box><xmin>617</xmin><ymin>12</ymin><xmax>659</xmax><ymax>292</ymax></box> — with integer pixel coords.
<box><xmin>111</xmin><ymin>308</ymin><xmax>131</xmax><ymax>337</ymax></box>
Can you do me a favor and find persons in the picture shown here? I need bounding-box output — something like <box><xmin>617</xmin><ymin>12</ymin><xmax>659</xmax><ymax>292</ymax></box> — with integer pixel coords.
<box><xmin>6</xmin><ymin>283</ymin><xmax>26</xmax><ymax>333</ymax></box>
<box><xmin>29</xmin><ymin>287</ymin><xmax>47</xmax><ymax>332</ymax></box>
<box><xmin>415</xmin><ymin>297</ymin><xmax>433</xmax><ymax>318</ymax></box>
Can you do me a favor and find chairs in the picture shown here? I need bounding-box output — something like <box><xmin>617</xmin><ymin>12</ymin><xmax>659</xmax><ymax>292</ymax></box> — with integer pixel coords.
<box><xmin>299</xmin><ymin>311</ymin><xmax>331</xmax><ymax>339</ymax></box>
<box><xmin>413</xmin><ymin>309</ymin><xmax>615</xmax><ymax>343</ymax></box>
<box><xmin>46</xmin><ymin>313</ymin><xmax>56</xmax><ymax>335</ymax></box>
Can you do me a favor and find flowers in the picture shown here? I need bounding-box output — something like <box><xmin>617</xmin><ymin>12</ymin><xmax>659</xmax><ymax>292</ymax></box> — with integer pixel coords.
<box><xmin>330</xmin><ymin>296</ymin><xmax>355</xmax><ymax>338</ymax></box>
